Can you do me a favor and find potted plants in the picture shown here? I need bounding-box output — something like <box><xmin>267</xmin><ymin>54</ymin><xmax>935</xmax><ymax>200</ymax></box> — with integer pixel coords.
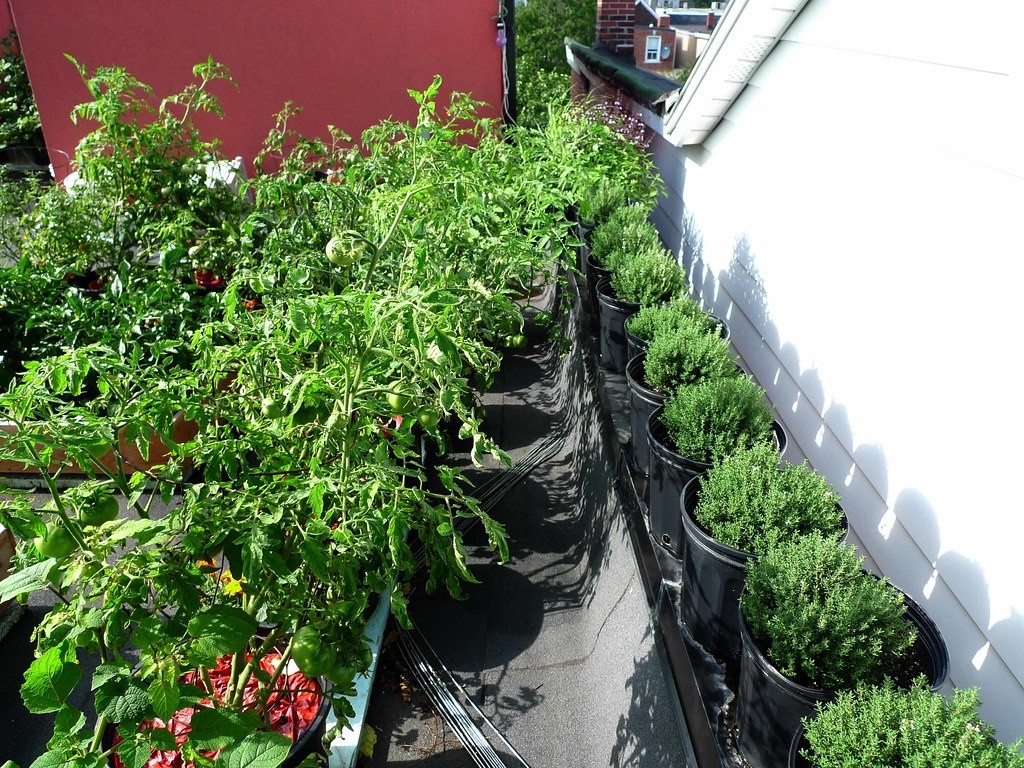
<box><xmin>0</xmin><ymin>26</ymin><xmax>1024</xmax><ymax>768</ymax></box>
